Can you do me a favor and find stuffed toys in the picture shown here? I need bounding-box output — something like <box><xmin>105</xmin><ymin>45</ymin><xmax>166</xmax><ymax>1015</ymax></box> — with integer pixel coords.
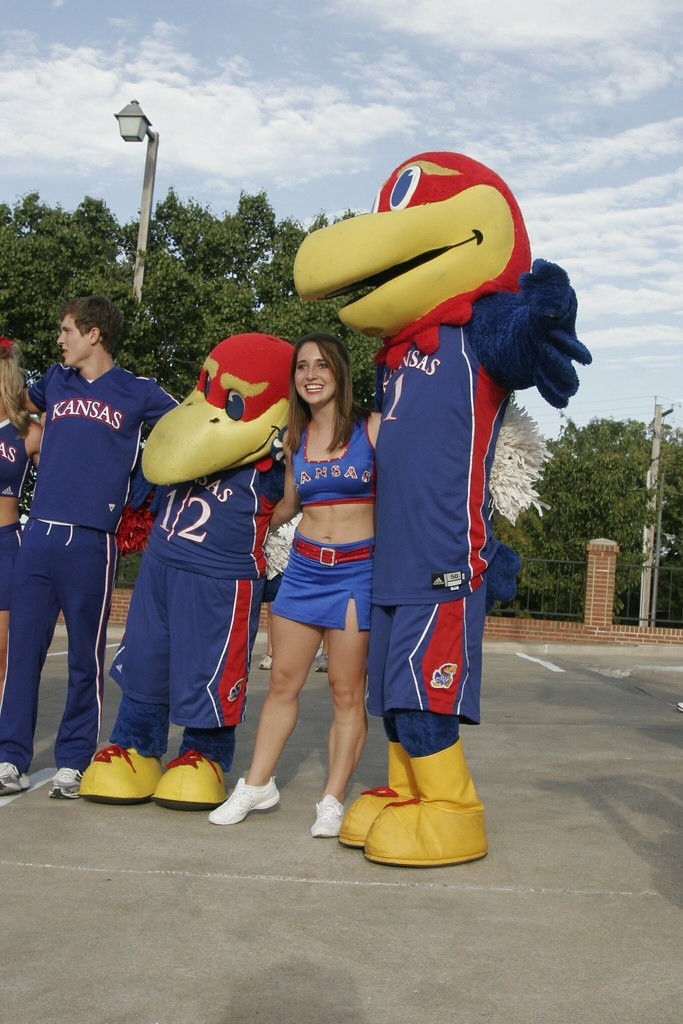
<box><xmin>294</xmin><ymin>152</ymin><xmax>592</xmax><ymax>866</ymax></box>
<box><xmin>77</xmin><ymin>333</ymin><xmax>295</xmax><ymax>812</ymax></box>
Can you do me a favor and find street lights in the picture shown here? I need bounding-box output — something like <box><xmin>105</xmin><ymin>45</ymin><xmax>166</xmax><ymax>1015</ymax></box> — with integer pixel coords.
<box><xmin>111</xmin><ymin>99</ymin><xmax>162</xmax><ymax>327</ymax></box>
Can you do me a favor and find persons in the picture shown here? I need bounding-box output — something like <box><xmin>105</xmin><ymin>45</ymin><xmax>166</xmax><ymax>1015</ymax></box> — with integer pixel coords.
<box><xmin>261</xmin><ymin>573</ymin><xmax>329</xmax><ymax>672</ymax></box>
<box><xmin>209</xmin><ymin>333</ymin><xmax>382</xmax><ymax>837</ymax></box>
<box><xmin>0</xmin><ymin>295</ymin><xmax>180</xmax><ymax>799</ymax></box>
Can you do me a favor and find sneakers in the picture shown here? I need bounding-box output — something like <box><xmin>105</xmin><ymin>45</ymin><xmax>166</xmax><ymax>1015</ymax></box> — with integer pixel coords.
<box><xmin>315</xmin><ymin>656</ymin><xmax>328</xmax><ymax>672</ymax></box>
<box><xmin>258</xmin><ymin>654</ymin><xmax>273</xmax><ymax>670</ymax></box>
<box><xmin>310</xmin><ymin>794</ymin><xmax>344</xmax><ymax>837</ymax></box>
<box><xmin>208</xmin><ymin>775</ymin><xmax>279</xmax><ymax>825</ymax></box>
<box><xmin>49</xmin><ymin>766</ymin><xmax>84</xmax><ymax>798</ymax></box>
<box><xmin>0</xmin><ymin>762</ymin><xmax>30</xmax><ymax>795</ymax></box>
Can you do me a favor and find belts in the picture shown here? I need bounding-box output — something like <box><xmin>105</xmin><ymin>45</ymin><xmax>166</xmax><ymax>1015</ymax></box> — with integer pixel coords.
<box><xmin>292</xmin><ymin>537</ymin><xmax>374</xmax><ymax>566</ymax></box>
